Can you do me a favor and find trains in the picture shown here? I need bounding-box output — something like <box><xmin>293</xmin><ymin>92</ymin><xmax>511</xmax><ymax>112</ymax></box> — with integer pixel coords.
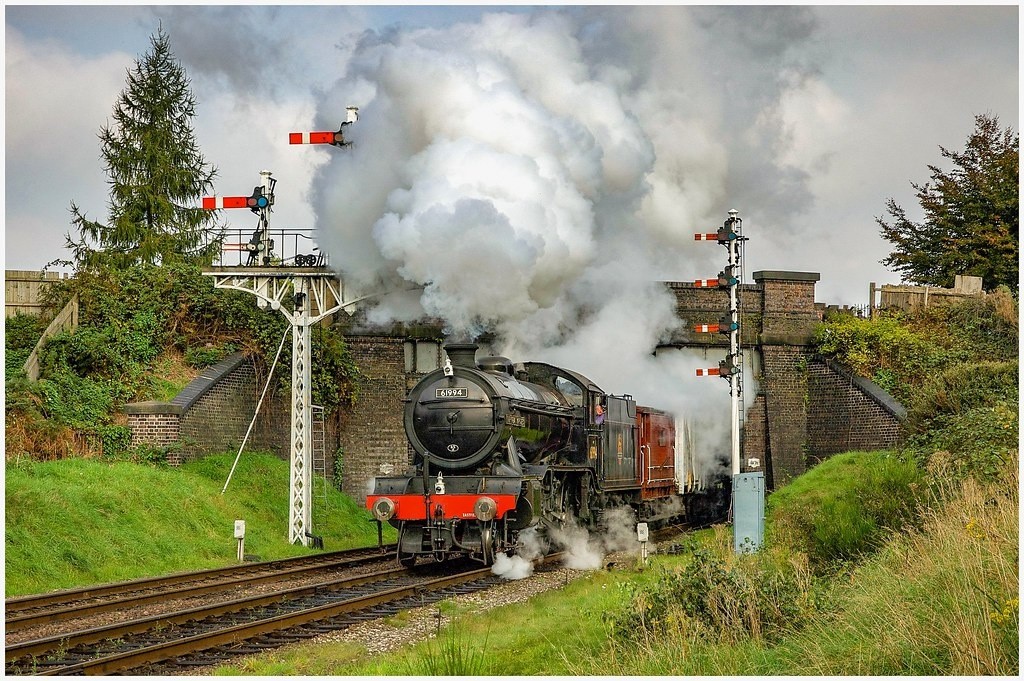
<box><xmin>366</xmin><ymin>342</ymin><xmax>733</xmax><ymax>572</ymax></box>
